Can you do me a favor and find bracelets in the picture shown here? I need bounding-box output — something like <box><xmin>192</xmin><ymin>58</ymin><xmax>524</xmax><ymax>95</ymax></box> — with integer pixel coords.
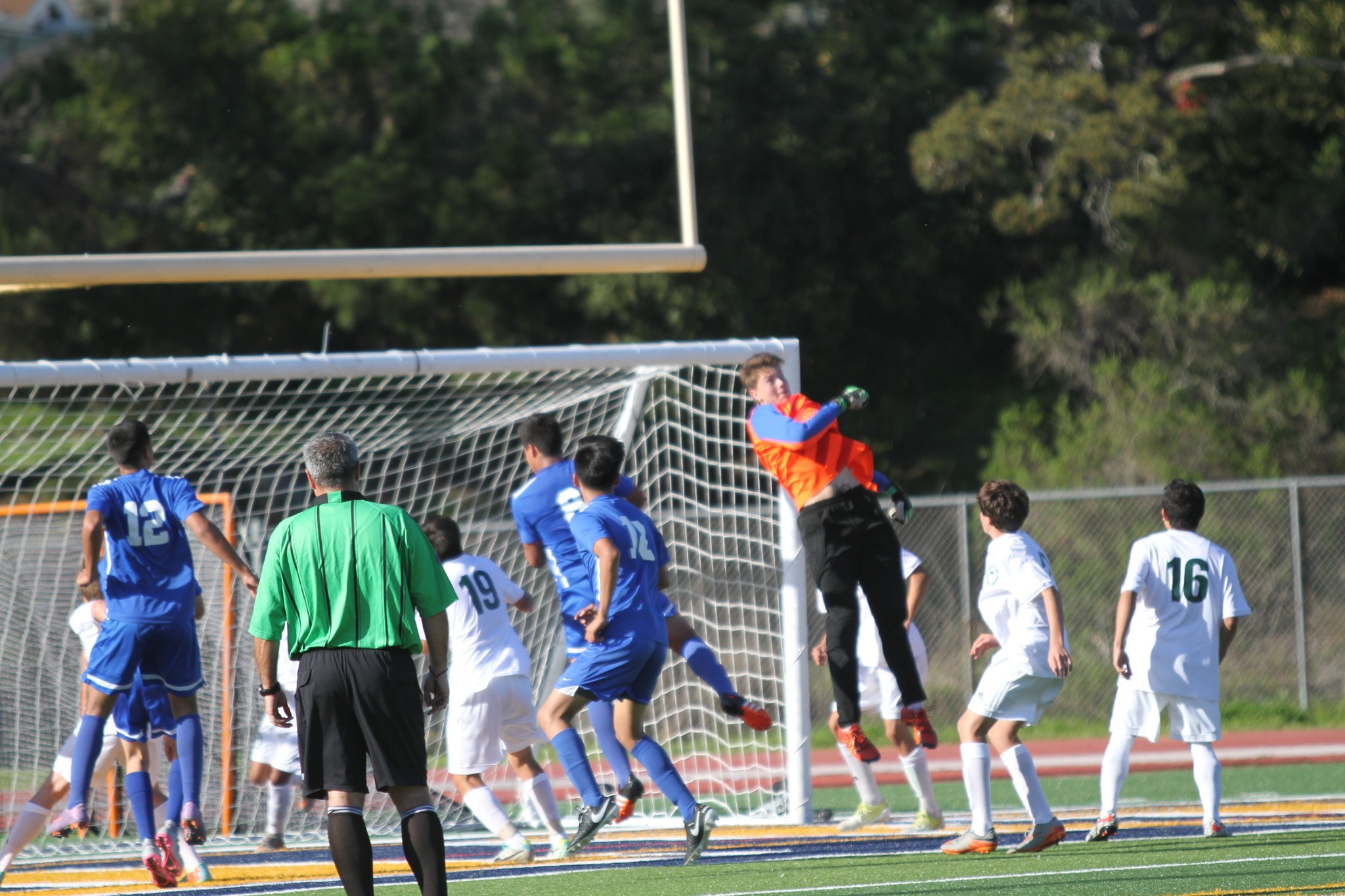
<box><xmin>429</xmin><ymin>665</ymin><xmax>448</xmax><ymax>678</ymax></box>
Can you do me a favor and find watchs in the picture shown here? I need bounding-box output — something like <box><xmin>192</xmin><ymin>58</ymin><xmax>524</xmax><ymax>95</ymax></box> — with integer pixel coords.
<box><xmin>256</xmin><ymin>681</ymin><xmax>281</xmax><ymax>697</ymax></box>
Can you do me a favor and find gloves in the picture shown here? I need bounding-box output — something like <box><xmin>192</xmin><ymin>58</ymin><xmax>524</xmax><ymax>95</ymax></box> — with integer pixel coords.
<box><xmin>835</xmin><ymin>385</ymin><xmax>870</xmax><ymax>413</ymax></box>
<box><xmin>886</xmin><ymin>482</ymin><xmax>913</xmax><ymax>526</ymax></box>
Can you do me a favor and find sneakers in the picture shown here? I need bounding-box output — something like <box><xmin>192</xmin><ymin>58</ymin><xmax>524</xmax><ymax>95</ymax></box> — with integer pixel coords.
<box><xmin>566</xmin><ymin>794</ymin><xmax>620</xmax><ymax>853</ymax></box>
<box><xmin>900</xmin><ymin>705</ymin><xmax>938</xmax><ymax>749</ymax></box>
<box><xmin>1085</xmin><ymin>810</ymin><xmax>1118</xmax><ymax>842</ymax></box>
<box><xmin>721</xmin><ymin>692</ymin><xmax>773</xmax><ymax>732</ymax></box>
<box><xmin>493</xmin><ymin>839</ymin><xmax>536</xmax><ymax>863</ymax></box>
<box><xmin>249</xmin><ymin>833</ymin><xmax>285</xmax><ymax>854</ymax></box>
<box><xmin>546</xmin><ymin>839</ymin><xmax>575</xmax><ymax>860</ymax></box>
<box><xmin>941</xmin><ymin>826</ymin><xmax>998</xmax><ymax>855</ymax></box>
<box><xmin>0</xmin><ymin>871</ymin><xmax>6</xmax><ymax>887</ymax></box>
<box><xmin>1006</xmin><ymin>816</ymin><xmax>1067</xmax><ymax>855</ymax></box>
<box><xmin>683</xmin><ymin>803</ymin><xmax>720</xmax><ymax>866</ymax></box>
<box><xmin>175</xmin><ymin>862</ymin><xmax>212</xmax><ymax>883</ymax></box>
<box><xmin>142</xmin><ymin>838</ymin><xmax>179</xmax><ymax>889</ymax></box>
<box><xmin>838</xmin><ymin>723</ymin><xmax>880</xmax><ymax>763</ymax></box>
<box><xmin>612</xmin><ymin>771</ymin><xmax>645</xmax><ymax>824</ymax></box>
<box><xmin>46</xmin><ymin>803</ymin><xmax>90</xmax><ymax>839</ymax></box>
<box><xmin>300</xmin><ymin>783</ymin><xmax>314</xmax><ymax>813</ymax></box>
<box><xmin>154</xmin><ymin>826</ymin><xmax>185</xmax><ymax>878</ymax></box>
<box><xmin>1204</xmin><ymin>818</ymin><xmax>1233</xmax><ymax>838</ymax></box>
<box><xmin>838</xmin><ymin>799</ymin><xmax>891</xmax><ymax>832</ymax></box>
<box><xmin>181</xmin><ymin>801</ymin><xmax>207</xmax><ymax>847</ymax></box>
<box><xmin>912</xmin><ymin>810</ymin><xmax>944</xmax><ymax>832</ymax></box>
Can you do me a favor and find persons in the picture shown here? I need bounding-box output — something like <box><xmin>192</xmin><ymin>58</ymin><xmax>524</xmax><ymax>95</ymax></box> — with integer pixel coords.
<box><xmin>420</xmin><ymin>510</ymin><xmax>573</xmax><ymax>863</ymax></box>
<box><xmin>1</xmin><ymin>569</ymin><xmax>212</xmax><ymax>882</ymax></box>
<box><xmin>939</xmin><ymin>480</ymin><xmax>1073</xmax><ymax>856</ymax></box>
<box><xmin>249</xmin><ymin>620</ymin><xmax>300</xmax><ymax>849</ymax></box>
<box><xmin>1084</xmin><ymin>478</ymin><xmax>1253</xmax><ymax>843</ymax></box>
<box><xmin>94</xmin><ymin>553</ymin><xmax>206</xmax><ymax>888</ymax></box>
<box><xmin>739</xmin><ymin>352</ymin><xmax>940</xmax><ymax>764</ymax></box>
<box><xmin>46</xmin><ymin>414</ymin><xmax>258</xmax><ymax>845</ymax></box>
<box><xmin>535</xmin><ymin>431</ymin><xmax>717</xmax><ymax>870</ymax></box>
<box><xmin>807</xmin><ymin>546</ymin><xmax>946</xmax><ymax>835</ymax></box>
<box><xmin>248</xmin><ymin>431</ymin><xmax>460</xmax><ymax>896</ymax></box>
<box><xmin>508</xmin><ymin>410</ymin><xmax>772</xmax><ymax>823</ymax></box>
